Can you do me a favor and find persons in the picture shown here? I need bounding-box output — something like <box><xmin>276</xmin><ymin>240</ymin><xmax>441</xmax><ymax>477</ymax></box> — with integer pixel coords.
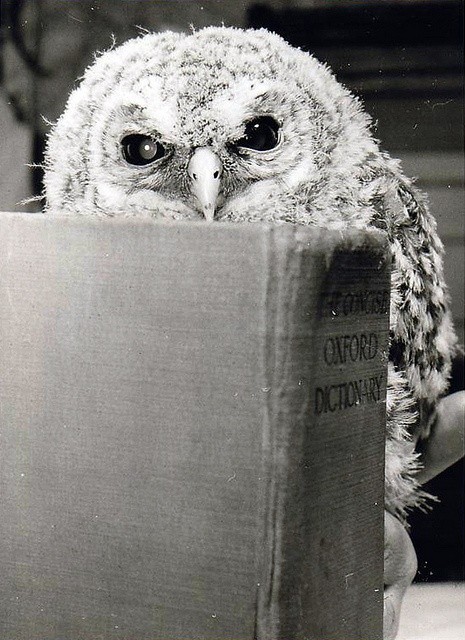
<box><xmin>383</xmin><ymin>389</ymin><xmax>465</xmax><ymax>639</ymax></box>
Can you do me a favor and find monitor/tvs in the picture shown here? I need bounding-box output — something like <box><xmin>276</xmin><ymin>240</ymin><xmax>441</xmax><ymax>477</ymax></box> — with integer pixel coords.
<box><xmin>0</xmin><ymin>211</ymin><xmax>393</xmax><ymax>639</ymax></box>
